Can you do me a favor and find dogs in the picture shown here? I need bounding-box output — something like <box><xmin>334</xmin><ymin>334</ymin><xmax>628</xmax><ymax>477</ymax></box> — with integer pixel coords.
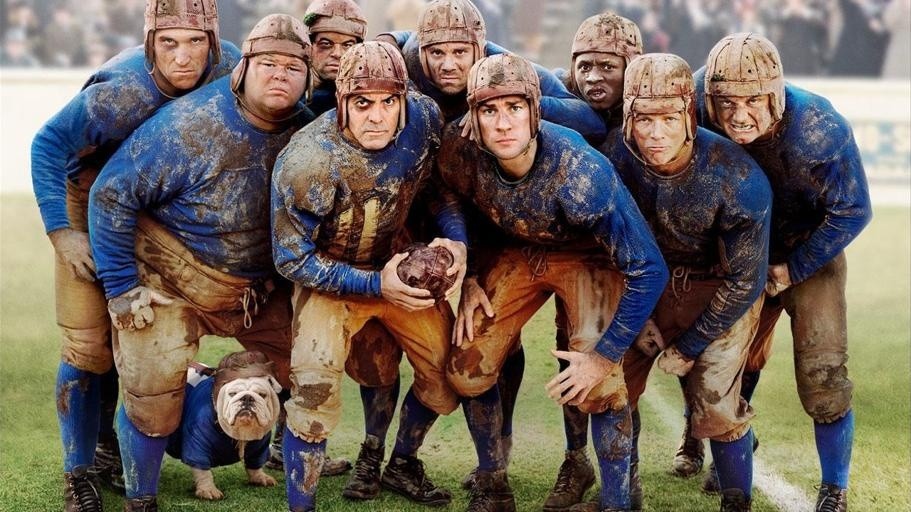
<box><xmin>165</xmin><ymin>362</ymin><xmax>278</xmax><ymax>501</ymax></box>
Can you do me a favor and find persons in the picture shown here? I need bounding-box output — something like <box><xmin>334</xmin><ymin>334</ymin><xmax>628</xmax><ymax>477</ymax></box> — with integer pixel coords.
<box><xmin>568</xmin><ymin>51</ymin><xmax>775</xmax><ymax>512</ymax></box>
<box><xmin>29</xmin><ymin>1</ymin><xmax>241</xmax><ymax>512</ymax></box>
<box><xmin>268</xmin><ymin>40</ymin><xmax>471</xmax><ymax>512</ymax></box>
<box><xmin>373</xmin><ymin>0</ymin><xmax>609</xmax><ymax>492</ymax></box>
<box><xmin>692</xmin><ymin>30</ymin><xmax>874</xmax><ymax>512</ymax></box>
<box><xmin>542</xmin><ymin>11</ymin><xmax>707</xmax><ymax>512</ymax></box>
<box><xmin>437</xmin><ymin>51</ymin><xmax>672</xmax><ymax>512</ymax></box>
<box><xmin>2</xmin><ymin>0</ymin><xmax>911</xmax><ymax>82</ymax></box>
<box><xmin>299</xmin><ymin>0</ymin><xmax>420</xmax><ymax>502</ymax></box>
<box><xmin>86</xmin><ymin>12</ymin><xmax>352</xmax><ymax>512</ymax></box>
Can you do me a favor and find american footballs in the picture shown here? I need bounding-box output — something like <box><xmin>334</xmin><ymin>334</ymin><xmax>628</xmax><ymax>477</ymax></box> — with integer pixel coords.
<box><xmin>396</xmin><ymin>242</ymin><xmax>456</xmax><ymax>303</ymax></box>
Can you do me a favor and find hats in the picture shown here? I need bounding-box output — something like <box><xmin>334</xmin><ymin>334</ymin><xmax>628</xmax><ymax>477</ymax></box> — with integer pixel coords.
<box><xmin>215</xmin><ymin>350</ymin><xmax>270</xmax><ymax>385</ymax></box>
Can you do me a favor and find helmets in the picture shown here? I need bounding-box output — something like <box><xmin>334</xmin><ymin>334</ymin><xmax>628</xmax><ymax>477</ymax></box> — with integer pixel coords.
<box><xmin>144</xmin><ymin>0</ymin><xmax>787</xmax><ymax>146</ymax></box>
